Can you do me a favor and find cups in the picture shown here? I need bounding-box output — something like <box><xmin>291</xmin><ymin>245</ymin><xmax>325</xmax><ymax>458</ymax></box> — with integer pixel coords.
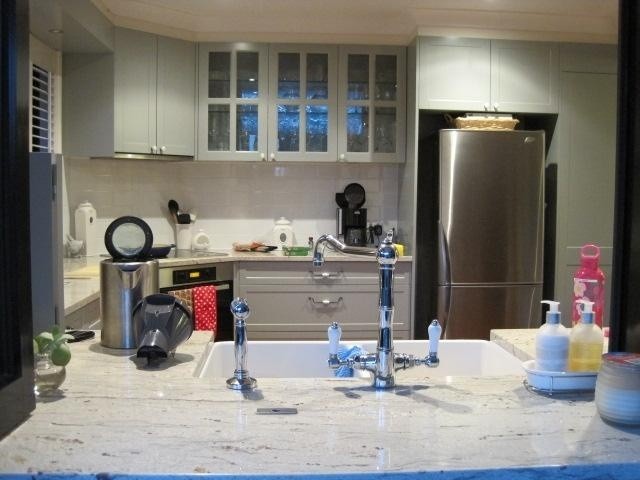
<box><xmin>175</xmin><ymin>223</ymin><xmax>194</xmax><ymax>250</ymax></box>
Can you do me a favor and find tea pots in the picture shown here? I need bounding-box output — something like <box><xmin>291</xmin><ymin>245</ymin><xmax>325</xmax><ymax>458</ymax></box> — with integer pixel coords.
<box><xmin>99</xmin><ymin>215</ymin><xmax>161</xmax><ymax>350</ymax></box>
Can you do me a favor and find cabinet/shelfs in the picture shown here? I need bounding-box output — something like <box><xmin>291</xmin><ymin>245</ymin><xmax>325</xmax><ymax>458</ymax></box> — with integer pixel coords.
<box><xmin>198</xmin><ymin>41</ymin><xmax>408</xmax><ymax>163</ymax></box>
<box><xmin>114</xmin><ymin>26</ymin><xmax>197</xmax><ymax>164</ymax></box>
<box><xmin>416</xmin><ymin>36</ymin><xmax>555</xmax><ymax>112</ymax></box>
<box><xmin>234</xmin><ymin>262</ymin><xmax>412</xmax><ymax>340</ymax></box>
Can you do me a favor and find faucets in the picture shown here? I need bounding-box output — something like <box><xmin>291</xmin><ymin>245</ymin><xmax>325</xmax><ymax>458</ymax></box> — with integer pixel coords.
<box><xmin>313</xmin><ymin>229</ymin><xmax>399</xmax><ymax>388</ymax></box>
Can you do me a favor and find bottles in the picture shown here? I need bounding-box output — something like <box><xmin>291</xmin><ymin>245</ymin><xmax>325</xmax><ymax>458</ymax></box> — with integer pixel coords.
<box><xmin>272</xmin><ymin>216</ymin><xmax>293</xmax><ymax>251</ymax></box>
<box><xmin>571</xmin><ymin>245</ymin><xmax>603</xmax><ymax>328</ymax></box>
<box><xmin>593</xmin><ymin>350</ymin><xmax>640</xmax><ymax>430</ymax></box>
<box><xmin>72</xmin><ymin>198</ymin><xmax>98</xmax><ymax>257</ymax></box>
<box><xmin>306</xmin><ymin>236</ymin><xmax>314</xmax><ymax>251</ymax></box>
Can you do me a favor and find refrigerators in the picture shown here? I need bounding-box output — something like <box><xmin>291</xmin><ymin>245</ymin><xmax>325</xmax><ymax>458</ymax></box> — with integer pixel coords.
<box><xmin>425</xmin><ymin>128</ymin><xmax>546</xmax><ymax>340</ymax></box>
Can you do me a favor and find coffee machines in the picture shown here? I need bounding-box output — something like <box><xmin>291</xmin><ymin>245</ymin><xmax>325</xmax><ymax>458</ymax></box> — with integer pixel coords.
<box><xmin>335</xmin><ymin>207</ymin><xmax>368</xmax><ymax>247</ymax></box>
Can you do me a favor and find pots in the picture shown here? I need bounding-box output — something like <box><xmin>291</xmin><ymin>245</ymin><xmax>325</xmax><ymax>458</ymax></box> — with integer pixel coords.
<box><xmin>150</xmin><ymin>243</ymin><xmax>176</xmax><ymax>258</ymax></box>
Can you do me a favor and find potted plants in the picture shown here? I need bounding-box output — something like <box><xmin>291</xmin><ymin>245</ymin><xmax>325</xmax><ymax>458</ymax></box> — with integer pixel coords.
<box><xmin>33</xmin><ymin>325</ymin><xmax>73</xmax><ymax>394</ymax></box>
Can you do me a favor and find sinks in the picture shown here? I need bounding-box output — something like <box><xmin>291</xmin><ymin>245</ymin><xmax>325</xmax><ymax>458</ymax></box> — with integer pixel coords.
<box><xmin>362</xmin><ymin>340</ymin><xmax>528</xmax><ymax>378</ymax></box>
<box><xmin>198</xmin><ymin>340</ymin><xmax>360</xmax><ymax>379</ymax></box>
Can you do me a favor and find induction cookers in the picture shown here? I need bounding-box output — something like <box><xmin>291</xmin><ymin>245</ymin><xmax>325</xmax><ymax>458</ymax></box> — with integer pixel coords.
<box><xmin>162</xmin><ymin>250</ymin><xmax>232</xmax><ymax>259</ymax></box>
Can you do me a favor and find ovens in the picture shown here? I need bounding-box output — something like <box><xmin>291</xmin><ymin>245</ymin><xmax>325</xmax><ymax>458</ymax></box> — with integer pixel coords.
<box><xmin>159</xmin><ymin>262</ymin><xmax>233</xmax><ymax>342</ymax></box>
